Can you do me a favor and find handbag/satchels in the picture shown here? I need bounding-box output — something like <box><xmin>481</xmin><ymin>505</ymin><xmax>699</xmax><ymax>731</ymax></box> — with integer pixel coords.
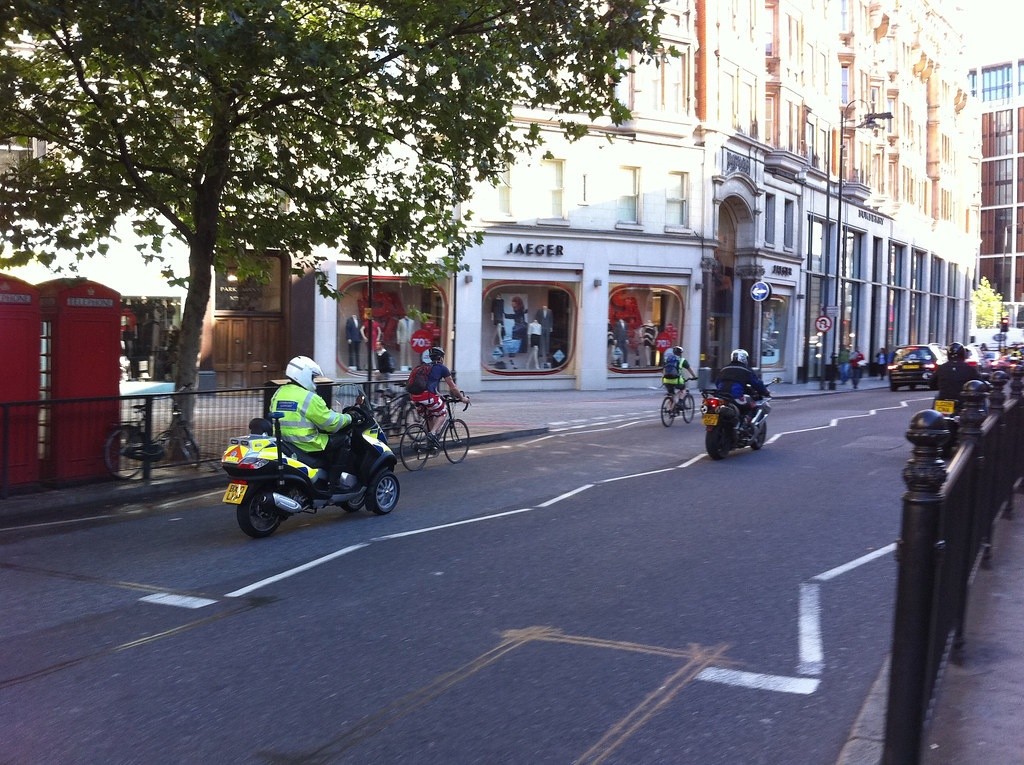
<box><xmin>388</xmin><ymin>356</ymin><xmax>396</xmax><ymax>374</ymax></box>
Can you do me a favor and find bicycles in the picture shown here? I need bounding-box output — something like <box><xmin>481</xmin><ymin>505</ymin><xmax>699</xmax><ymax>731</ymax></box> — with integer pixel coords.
<box><xmin>370</xmin><ymin>384</ymin><xmax>428</xmax><ymax>441</ymax></box>
<box><xmin>399</xmin><ymin>391</ymin><xmax>470</xmax><ymax>472</ymax></box>
<box><xmin>105</xmin><ymin>383</ymin><xmax>201</xmax><ymax>479</ymax></box>
<box><xmin>661</xmin><ymin>377</ymin><xmax>697</xmax><ymax>428</ymax></box>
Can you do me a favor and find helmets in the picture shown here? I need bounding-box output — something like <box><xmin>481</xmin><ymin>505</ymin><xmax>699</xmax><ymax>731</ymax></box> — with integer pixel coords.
<box><xmin>947</xmin><ymin>343</ymin><xmax>966</xmax><ymax>362</ymax></box>
<box><xmin>730</xmin><ymin>349</ymin><xmax>749</xmax><ymax>368</ymax></box>
<box><xmin>673</xmin><ymin>346</ymin><xmax>684</xmax><ymax>353</ymax></box>
<box><xmin>286</xmin><ymin>355</ymin><xmax>323</xmax><ymax>392</ymax></box>
<box><xmin>429</xmin><ymin>347</ymin><xmax>445</xmax><ymax>357</ymax></box>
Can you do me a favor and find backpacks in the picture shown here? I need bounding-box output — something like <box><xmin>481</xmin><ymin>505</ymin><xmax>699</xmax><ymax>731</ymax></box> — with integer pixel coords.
<box><xmin>406</xmin><ymin>362</ymin><xmax>435</xmax><ymax>395</ymax></box>
<box><xmin>664</xmin><ymin>355</ymin><xmax>681</xmax><ymax>379</ymax></box>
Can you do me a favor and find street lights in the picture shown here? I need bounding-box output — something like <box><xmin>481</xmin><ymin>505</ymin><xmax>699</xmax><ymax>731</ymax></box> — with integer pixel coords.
<box><xmin>829</xmin><ymin>100</ymin><xmax>876</xmax><ymax>391</ymax></box>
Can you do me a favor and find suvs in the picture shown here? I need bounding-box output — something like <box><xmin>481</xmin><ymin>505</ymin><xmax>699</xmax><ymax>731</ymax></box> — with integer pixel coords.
<box><xmin>888</xmin><ymin>342</ymin><xmax>947</xmax><ymax>390</ymax></box>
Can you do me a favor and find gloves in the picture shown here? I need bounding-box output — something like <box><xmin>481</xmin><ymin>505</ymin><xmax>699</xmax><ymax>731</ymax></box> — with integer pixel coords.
<box><xmin>347</xmin><ymin>410</ymin><xmax>365</xmax><ymax>423</ymax></box>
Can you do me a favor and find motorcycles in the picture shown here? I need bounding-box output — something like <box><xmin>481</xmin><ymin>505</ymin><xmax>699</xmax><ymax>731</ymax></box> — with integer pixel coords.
<box><xmin>221</xmin><ymin>383</ymin><xmax>399</xmax><ymax>539</ymax></box>
<box><xmin>933</xmin><ymin>374</ymin><xmax>988</xmax><ymax>420</ymax></box>
<box><xmin>700</xmin><ymin>377</ymin><xmax>781</xmax><ymax>459</ymax></box>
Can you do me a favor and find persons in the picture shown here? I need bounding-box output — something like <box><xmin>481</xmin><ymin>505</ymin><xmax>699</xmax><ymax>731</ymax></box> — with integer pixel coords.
<box><xmin>607</xmin><ymin>317</ymin><xmax>616</xmax><ymax>367</ymax></box>
<box><xmin>359</xmin><ymin>315</ymin><xmax>382</xmax><ymax>370</ymax></box>
<box><xmin>374</xmin><ymin>340</ymin><xmax>395</xmax><ymax>408</ymax></box>
<box><xmin>269</xmin><ymin>355</ymin><xmax>363</xmax><ymax>494</ymax></box>
<box><xmin>535</xmin><ymin>306</ymin><xmax>553</xmax><ymax>356</ymax></box>
<box><xmin>406</xmin><ymin>346</ymin><xmax>470</xmax><ymax>453</ymax></box>
<box><xmin>345</xmin><ymin>315</ymin><xmax>362</xmax><ymax>371</ymax></box>
<box><xmin>836</xmin><ymin>343</ymin><xmax>895</xmax><ymax>389</ymax></box>
<box><xmin>716</xmin><ymin>348</ymin><xmax>771</xmax><ymax>439</ymax></box>
<box><xmin>614</xmin><ymin>318</ymin><xmax>630</xmax><ymax>364</ymax></box>
<box><xmin>661</xmin><ymin>346</ymin><xmax>696</xmax><ymax>417</ymax></box>
<box><xmin>527</xmin><ymin>319</ymin><xmax>542</xmax><ymax>370</ymax></box>
<box><xmin>931</xmin><ymin>342</ymin><xmax>981</xmax><ymax>418</ymax></box>
<box><xmin>397</xmin><ymin>316</ymin><xmax>415</xmax><ymax>370</ymax></box>
<box><xmin>640</xmin><ymin>319</ymin><xmax>658</xmax><ymax>367</ymax></box>
<box><xmin>490</xmin><ymin>292</ymin><xmax>506</xmax><ymax>345</ymax></box>
<box><xmin>492</xmin><ymin>320</ymin><xmax>506</xmax><ymax>370</ymax></box>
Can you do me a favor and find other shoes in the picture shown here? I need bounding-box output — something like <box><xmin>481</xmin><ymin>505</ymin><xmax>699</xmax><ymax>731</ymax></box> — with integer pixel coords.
<box><xmin>427</xmin><ymin>432</ymin><xmax>442</xmax><ymax>449</ymax></box>
<box><xmin>386</xmin><ymin>400</ymin><xmax>390</xmax><ymax>403</ymax></box>
<box><xmin>370</xmin><ymin>402</ymin><xmax>378</xmax><ymax>406</ymax></box>
<box><xmin>314</xmin><ymin>478</ymin><xmax>328</xmax><ymax>492</ymax></box>
<box><xmin>739</xmin><ymin>423</ymin><xmax>750</xmax><ymax>433</ymax></box>
<box><xmin>675</xmin><ymin>402</ymin><xmax>685</xmax><ymax>408</ymax></box>
<box><xmin>327</xmin><ymin>480</ymin><xmax>352</xmax><ymax>493</ymax></box>
<box><xmin>670</xmin><ymin>413</ymin><xmax>677</xmax><ymax>416</ymax></box>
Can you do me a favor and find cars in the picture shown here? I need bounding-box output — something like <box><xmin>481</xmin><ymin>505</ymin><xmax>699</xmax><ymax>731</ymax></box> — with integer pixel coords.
<box><xmin>964</xmin><ymin>346</ymin><xmax>1000</xmax><ymax>369</ymax></box>
